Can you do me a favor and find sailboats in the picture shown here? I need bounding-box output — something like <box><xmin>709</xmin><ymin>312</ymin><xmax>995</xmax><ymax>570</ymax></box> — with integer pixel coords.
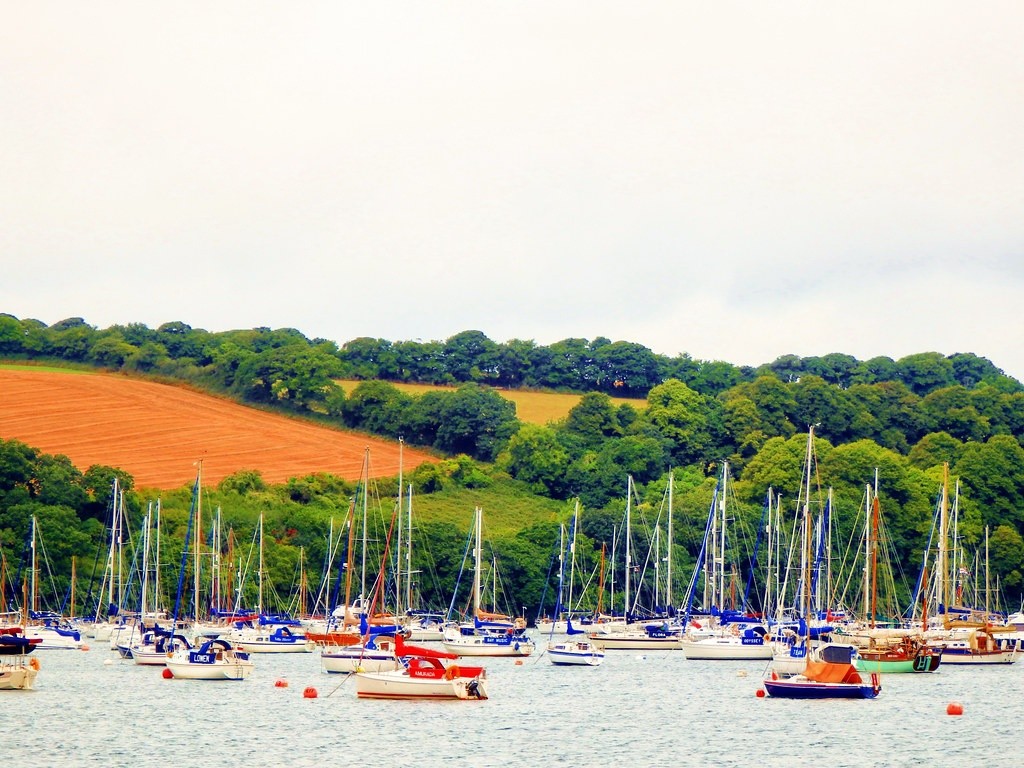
<box><xmin>0</xmin><ymin>426</ymin><xmax>1024</xmax><ymax>704</ymax></box>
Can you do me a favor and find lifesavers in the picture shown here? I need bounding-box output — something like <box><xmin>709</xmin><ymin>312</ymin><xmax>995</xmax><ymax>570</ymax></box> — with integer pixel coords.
<box><xmin>30</xmin><ymin>658</ymin><xmax>39</xmax><ymax>671</ymax></box>
<box><xmin>764</xmin><ymin>634</ymin><xmax>771</xmax><ymax>642</ymax></box>
